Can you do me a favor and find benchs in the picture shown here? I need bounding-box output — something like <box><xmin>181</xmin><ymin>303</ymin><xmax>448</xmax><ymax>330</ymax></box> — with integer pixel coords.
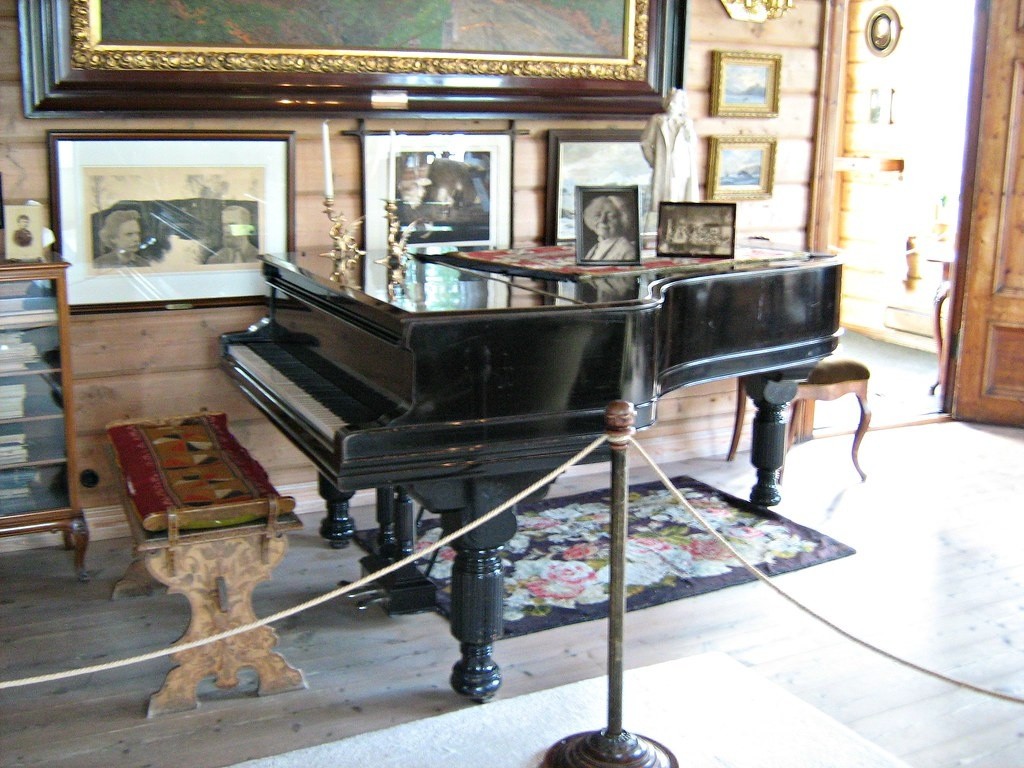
<box><xmin>98</xmin><ymin>408</ymin><xmax>310</xmax><ymax>719</ymax></box>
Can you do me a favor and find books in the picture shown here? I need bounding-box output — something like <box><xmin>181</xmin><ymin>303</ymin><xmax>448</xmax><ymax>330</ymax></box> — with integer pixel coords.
<box><xmin>0</xmin><ymin>280</ymin><xmax>57</xmax><ymax>513</ymax></box>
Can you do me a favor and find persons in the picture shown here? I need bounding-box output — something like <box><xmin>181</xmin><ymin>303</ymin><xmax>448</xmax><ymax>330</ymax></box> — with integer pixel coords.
<box><xmin>93</xmin><ymin>210</ymin><xmax>149</xmax><ymax>267</ymax></box>
<box><xmin>204</xmin><ymin>205</ymin><xmax>258</xmax><ymax>264</ymax></box>
<box><xmin>644</xmin><ymin>87</ymin><xmax>701</xmax><ymax>249</ymax></box>
<box><xmin>582</xmin><ymin>196</ymin><xmax>636</xmax><ymax>262</ymax></box>
<box><xmin>12</xmin><ymin>214</ymin><xmax>31</xmax><ymax>245</ymax></box>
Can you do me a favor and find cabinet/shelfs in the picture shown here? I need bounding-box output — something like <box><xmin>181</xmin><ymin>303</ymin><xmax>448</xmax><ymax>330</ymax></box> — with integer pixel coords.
<box><xmin>0</xmin><ymin>251</ymin><xmax>89</xmax><ymax>581</ymax></box>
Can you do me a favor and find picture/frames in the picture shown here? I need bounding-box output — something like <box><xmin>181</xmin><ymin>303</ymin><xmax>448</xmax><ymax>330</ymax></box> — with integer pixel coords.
<box><xmin>711</xmin><ymin>48</ymin><xmax>782</xmax><ymax>118</ymax></box>
<box><xmin>362</xmin><ymin>246</ymin><xmax>514</xmax><ymax>312</ymax></box>
<box><xmin>3</xmin><ymin>205</ymin><xmax>44</xmax><ymax>264</ymax></box>
<box><xmin>340</xmin><ymin>119</ymin><xmax>529</xmax><ymax>245</ymax></box>
<box><xmin>543</xmin><ymin>277</ymin><xmax>659</xmax><ymax>305</ymax></box>
<box><xmin>18</xmin><ymin>0</ymin><xmax>687</xmax><ymax>119</ymax></box>
<box><xmin>704</xmin><ymin>135</ymin><xmax>778</xmax><ymax>201</ymax></box>
<box><xmin>574</xmin><ymin>184</ymin><xmax>641</xmax><ymax>266</ymax></box>
<box><xmin>546</xmin><ymin>128</ymin><xmax>656</xmax><ymax>245</ymax></box>
<box><xmin>657</xmin><ymin>201</ymin><xmax>736</xmax><ymax>260</ymax></box>
<box><xmin>46</xmin><ymin>129</ymin><xmax>296</xmax><ymax>314</ymax></box>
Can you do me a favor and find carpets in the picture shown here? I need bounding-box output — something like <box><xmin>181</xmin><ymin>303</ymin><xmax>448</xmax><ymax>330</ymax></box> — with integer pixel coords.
<box><xmin>414</xmin><ymin>244</ymin><xmax>809</xmax><ymax>279</ymax></box>
<box><xmin>352</xmin><ymin>474</ymin><xmax>856</xmax><ymax>641</ymax></box>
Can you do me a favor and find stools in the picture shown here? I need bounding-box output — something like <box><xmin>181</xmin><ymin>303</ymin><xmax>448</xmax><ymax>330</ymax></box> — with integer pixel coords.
<box><xmin>726</xmin><ymin>358</ymin><xmax>872</xmax><ymax>481</ymax></box>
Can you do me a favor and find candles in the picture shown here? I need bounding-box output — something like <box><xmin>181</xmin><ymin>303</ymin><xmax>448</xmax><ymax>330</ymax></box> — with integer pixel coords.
<box><xmin>388</xmin><ymin>130</ymin><xmax>396</xmax><ymax>201</ymax></box>
<box><xmin>322</xmin><ymin>119</ymin><xmax>333</xmax><ymax>199</ymax></box>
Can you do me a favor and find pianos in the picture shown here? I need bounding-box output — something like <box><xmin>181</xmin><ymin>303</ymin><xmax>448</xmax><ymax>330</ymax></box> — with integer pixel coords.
<box><xmin>215</xmin><ymin>247</ymin><xmax>845</xmax><ymax>707</ymax></box>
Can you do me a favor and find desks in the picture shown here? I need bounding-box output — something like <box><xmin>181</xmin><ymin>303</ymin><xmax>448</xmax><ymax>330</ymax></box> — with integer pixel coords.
<box><xmin>926</xmin><ymin>255</ymin><xmax>954</xmax><ymax>396</ymax></box>
